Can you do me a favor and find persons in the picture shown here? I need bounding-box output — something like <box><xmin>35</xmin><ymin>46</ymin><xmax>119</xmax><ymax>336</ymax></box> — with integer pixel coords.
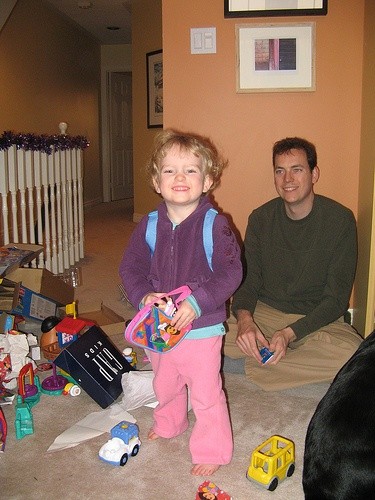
<box><xmin>220</xmin><ymin>137</ymin><xmax>364</xmax><ymax>392</ymax></box>
<box><xmin>118</xmin><ymin>127</ymin><xmax>243</xmax><ymax>477</ymax></box>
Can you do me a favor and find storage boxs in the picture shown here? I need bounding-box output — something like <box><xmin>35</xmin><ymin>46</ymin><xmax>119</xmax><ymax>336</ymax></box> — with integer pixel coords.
<box><xmin>0</xmin><ymin>268</ymin><xmax>72</xmax><ymax>323</ymax></box>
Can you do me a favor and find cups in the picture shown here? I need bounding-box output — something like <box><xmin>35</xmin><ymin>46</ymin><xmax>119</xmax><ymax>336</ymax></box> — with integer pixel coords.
<box><xmin>69</xmin><ymin>268</ymin><xmax>80</xmax><ymax>288</ymax></box>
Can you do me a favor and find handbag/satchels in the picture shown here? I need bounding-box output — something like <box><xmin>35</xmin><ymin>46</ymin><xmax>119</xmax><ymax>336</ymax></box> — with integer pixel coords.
<box><xmin>124</xmin><ymin>285</ymin><xmax>193</xmax><ymax>355</ymax></box>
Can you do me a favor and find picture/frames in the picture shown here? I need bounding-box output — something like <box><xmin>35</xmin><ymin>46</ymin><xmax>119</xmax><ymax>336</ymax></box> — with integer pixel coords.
<box><xmin>223</xmin><ymin>0</ymin><xmax>329</xmax><ymax>18</ymax></box>
<box><xmin>146</xmin><ymin>48</ymin><xmax>164</xmax><ymax>130</ymax></box>
<box><xmin>234</xmin><ymin>22</ymin><xmax>317</xmax><ymax>93</ymax></box>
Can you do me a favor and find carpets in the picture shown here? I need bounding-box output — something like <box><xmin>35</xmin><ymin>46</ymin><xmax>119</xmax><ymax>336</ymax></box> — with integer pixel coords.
<box><xmin>0</xmin><ymin>333</ymin><xmax>335</xmax><ymax>500</ymax></box>
